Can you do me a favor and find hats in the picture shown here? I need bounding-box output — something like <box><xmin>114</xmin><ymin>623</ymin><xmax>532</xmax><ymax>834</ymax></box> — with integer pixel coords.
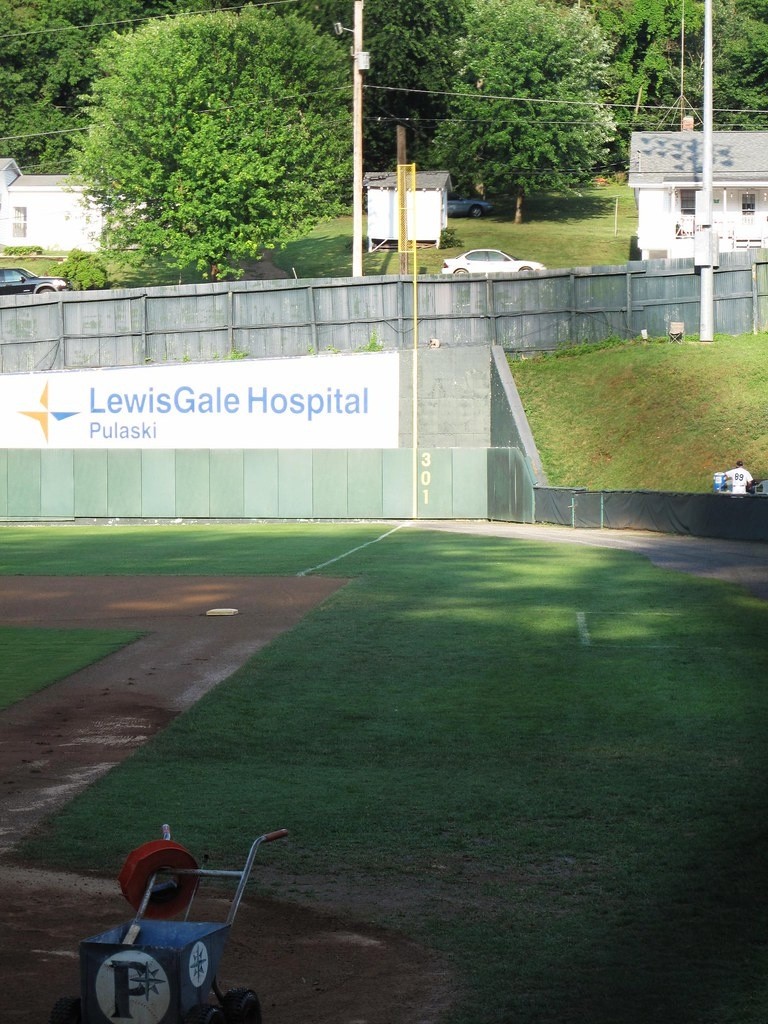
<box><xmin>736</xmin><ymin>461</ymin><xmax>743</xmax><ymax>466</ymax></box>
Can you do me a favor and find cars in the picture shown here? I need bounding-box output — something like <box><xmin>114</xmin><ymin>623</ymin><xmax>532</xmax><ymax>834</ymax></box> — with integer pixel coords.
<box><xmin>448</xmin><ymin>191</ymin><xmax>495</xmax><ymax>217</ymax></box>
<box><xmin>441</xmin><ymin>248</ymin><xmax>547</xmax><ymax>273</ymax></box>
<box><xmin>0</xmin><ymin>267</ymin><xmax>72</xmax><ymax>294</ymax></box>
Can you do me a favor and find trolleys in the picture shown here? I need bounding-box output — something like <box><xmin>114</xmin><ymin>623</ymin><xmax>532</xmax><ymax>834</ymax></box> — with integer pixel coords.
<box><xmin>50</xmin><ymin>822</ymin><xmax>291</xmax><ymax>1024</ymax></box>
